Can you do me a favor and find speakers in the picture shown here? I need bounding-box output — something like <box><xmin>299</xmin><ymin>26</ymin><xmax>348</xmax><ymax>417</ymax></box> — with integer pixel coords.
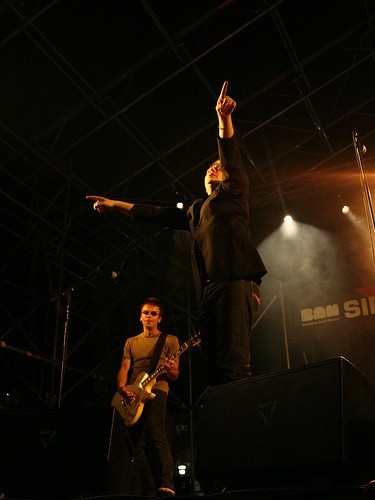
<box><xmin>0</xmin><ymin>406</ymin><xmax>143</xmax><ymax>500</ymax></box>
<box><xmin>194</xmin><ymin>357</ymin><xmax>375</xmax><ymax>500</ymax></box>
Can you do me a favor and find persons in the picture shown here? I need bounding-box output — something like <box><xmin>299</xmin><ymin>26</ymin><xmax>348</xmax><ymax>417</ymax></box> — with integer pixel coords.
<box><xmin>87</xmin><ymin>80</ymin><xmax>268</xmax><ymax>382</ymax></box>
<box><xmin>117</xmin><ymin>297</ymin><xmax>180</xmax><ymax>498</ymax></box>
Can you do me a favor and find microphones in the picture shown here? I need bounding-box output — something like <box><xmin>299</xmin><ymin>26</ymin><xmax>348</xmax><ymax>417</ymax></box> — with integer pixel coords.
<box><xmin>98</xmin><ymin>271</ymin><xmax>119</xmax><ymax>280</ymax></box>
<box><xmin>354</xmin><ymin>131</ymin><xmax>361</xmax><ymax>145</ymax></box>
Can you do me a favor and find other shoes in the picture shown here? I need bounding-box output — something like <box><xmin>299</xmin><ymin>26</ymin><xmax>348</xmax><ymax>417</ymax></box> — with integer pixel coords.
<box><xmin>158</xmin><ymin>481</ymin><xmax>175</xmax><ymax>497</ymax></box>
<box><xmin>194</xmin><ymin>370</ymin><xmax>232</xmax><ymax>385</ymax></box>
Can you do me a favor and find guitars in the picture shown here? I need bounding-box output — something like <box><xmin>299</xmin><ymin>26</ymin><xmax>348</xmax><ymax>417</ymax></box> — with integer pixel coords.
<box><xmin>109</xmin><ymin>333</ymin><xmax>202</xmax><ymax>424</ymax></box>
